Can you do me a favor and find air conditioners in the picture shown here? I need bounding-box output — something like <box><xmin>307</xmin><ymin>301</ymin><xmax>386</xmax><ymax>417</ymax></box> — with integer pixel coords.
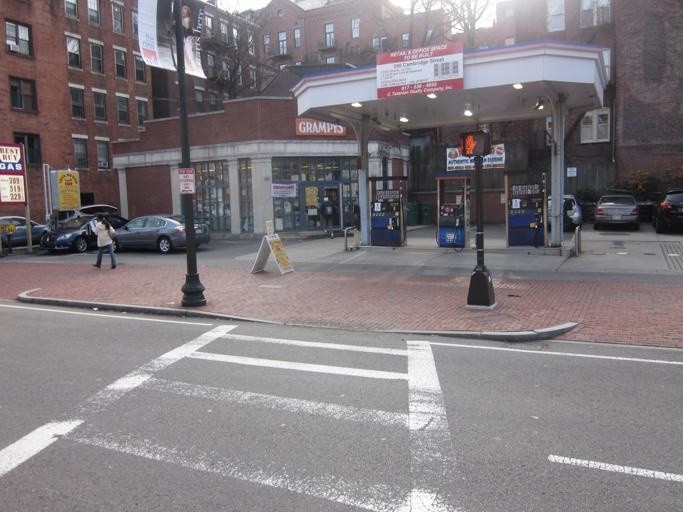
<box><xmin>98</xmin><ymin>161</ymin><xmax>108</xmax><ymax>168</ymax></box>
<box><xmin>8</xmin><ymin>44</ymin><xmax>19</xmax><ymax>53</ymax></box>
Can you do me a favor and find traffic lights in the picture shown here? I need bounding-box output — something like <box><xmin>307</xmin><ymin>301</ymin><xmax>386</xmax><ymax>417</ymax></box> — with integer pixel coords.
<box><xmin>460</xmin><ymin>130</ymin><xmax>491</xmax><ymax>156</ymax></box>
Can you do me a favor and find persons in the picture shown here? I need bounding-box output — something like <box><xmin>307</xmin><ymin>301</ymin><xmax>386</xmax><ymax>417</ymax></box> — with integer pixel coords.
<box><xmin>91</xmin><ymin>214</ymin><xmax>117</xmax><ymax>269</ymax></box>
<box><xmin>317</xmin><ymin>196</ymin><xmax>337</xmax><ymax>239</ymax></box>
<box><xmin>172</xmin><ymin>5</ymin><xmax>198</xmax><ymax>71</ymax></box>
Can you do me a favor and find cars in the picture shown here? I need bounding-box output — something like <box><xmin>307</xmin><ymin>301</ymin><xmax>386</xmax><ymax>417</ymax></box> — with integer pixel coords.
<box><xmin>114</xmin><ymin>214</ymin><xmax>211</xmax><ymax>254</ymax></box>
<box><xmin>594</xmin><ymin>192</ymin><xmax>640</xmax><ymax>232</ymax></box>
<box><xmin>540</xmin><ymin>191</ymin><xmax>584</xmax><ymax>230</ymax></box>
<box><xmin>0</xmin><ymin>204</ymin><xmax>130</xmax><ymax>259</ymax></box>
<box><xmin>651</xmin><ymin>186</ymin><xmax>682</xmax><ymax>236</ymax></box>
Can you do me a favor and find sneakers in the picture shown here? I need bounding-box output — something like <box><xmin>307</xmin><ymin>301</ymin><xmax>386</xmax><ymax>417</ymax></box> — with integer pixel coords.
<box><xmin>92</xmin><ymin>263</ymin><xmax>100</xmax><ymax>268</ymax></box>
<box><xmin>111</xmin><ymin>264</ymin><xmax>116</xmax><ymax>269</ymax></box>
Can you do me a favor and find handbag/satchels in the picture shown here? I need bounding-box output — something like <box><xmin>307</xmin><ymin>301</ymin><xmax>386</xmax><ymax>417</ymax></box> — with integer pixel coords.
<box><xmin>108</xmin><ymin>229</ymin><xmax>117</xmax><ymax>239</ymax></box>
